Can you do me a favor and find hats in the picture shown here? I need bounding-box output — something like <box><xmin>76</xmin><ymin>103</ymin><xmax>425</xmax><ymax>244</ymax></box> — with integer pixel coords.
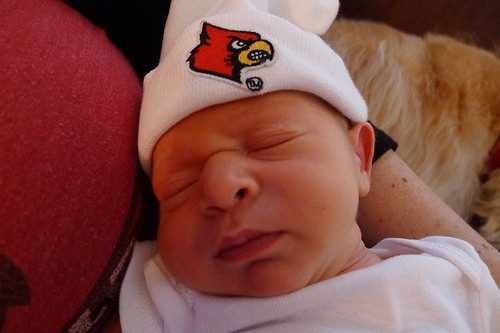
<box><xmin>138</xmin><ymin>0</ymin><xmax>369</xmax><ymax>174</ymax></box>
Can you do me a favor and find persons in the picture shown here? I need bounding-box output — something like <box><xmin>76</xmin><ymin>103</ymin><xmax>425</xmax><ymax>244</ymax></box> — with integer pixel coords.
<box><xmin>73</xmin><ymin>0</ymin><xmax>500</xmax><ymax>333</ymax></box>
